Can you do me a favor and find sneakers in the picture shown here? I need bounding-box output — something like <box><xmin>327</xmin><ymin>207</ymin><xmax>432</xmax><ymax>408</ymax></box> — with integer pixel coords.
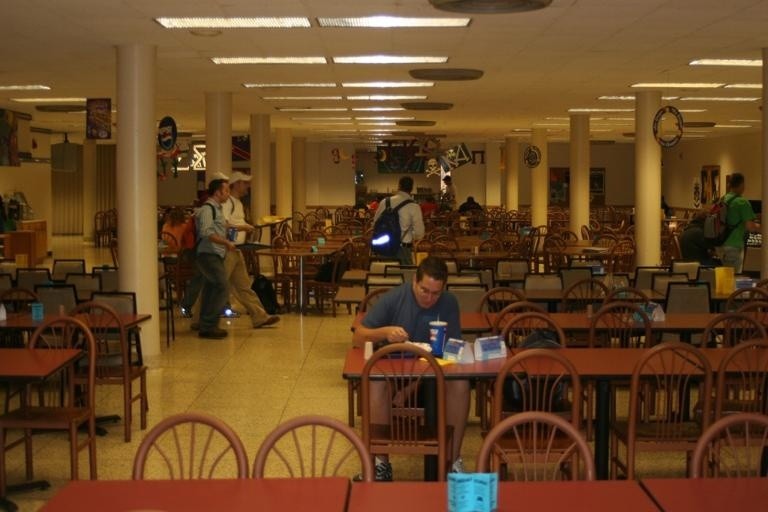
<box><xmin>224</xmin><ymin>309</ymin><xmax>240</xmax><ymax>318</ymax></box>
<box><xmin>178</xmin><ymin>301</ymin><xmax>192</xmax><ymax>318</ymax></box>
<box><xmin>254</xmin><ymin>316</ymin><xmax>280</xmax><ymax>329</ymax></box>
<box><xmin>352</xmin><ymin>462</ymin><xmax>391</xmax><ymax>482</ymax></box>
<box><xmin>198</xmin><ymin>327</ymin><xmax>227</xmax><ymax>339</ymax></box>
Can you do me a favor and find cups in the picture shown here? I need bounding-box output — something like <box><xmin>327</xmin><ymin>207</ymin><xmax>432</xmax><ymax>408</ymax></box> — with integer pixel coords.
<box><xmin>428</xmin><ymin>320</ymin><xmax>448</xmax><ymax>358</ymax></box>
<box><xmin>31</xmin><ymin>303</ymin><xmax>44</xmax><ymax>321</ymax></box>
<box><xmin>227</xmin><ymin>225</ymin><xmax>238</xmax><ymax>242</ymax></box>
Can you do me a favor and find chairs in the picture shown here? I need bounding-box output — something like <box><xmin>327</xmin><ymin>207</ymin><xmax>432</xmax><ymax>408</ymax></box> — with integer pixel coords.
<box><xmin>688</xmin><ymin>410</ymin><xmax>768</xmax><ymax>483</ymax></box>
<box><xmin>360</xmin><ymin>287</ymin><xmax>392</xmax><ymax>320</ymax></box>
<box><xmin>687</xmin><ymin>313</ymin><xmax>767</xmax><ymax>428</ymax></box>
<box><xmin>245</xmin><ymin>413</ymin><xmax>377</xmax><ymax>487</ymax></box>
<box><xmin>52</xmin><ymin>260</ymin><xmax>85</xmax><ymax>286</ymax></box>
<box><xmin>751</xmin><ymin>280</ymin><xmax>768</xmax><ymax>301</ymax></box>
<box><xmin>65</xmin><ymin>273</ymin><xmax>102</xmax><ymax>305</ymax></box>
<box><xmin>152</xmin><ymin>205</ymin><xmax>204</xmax><ymax>311</ymax></box>
<box><xmin>488</xmin><ymin>310</ymin><xmax>642</xmax><ymax>350</ymax></box>
<box><xmin>448</xmin><ymin>284</ymin><xmax>491</xmax><ymax>341</ymax></box>
<box><xmin>601</xmin><ymin>288</ymin><xmax>653</xmax><ymax>345</ymax></box>
<box><xmin>92</xmin><ymin>185</ymin><xmax>124</xmax><ymax>269</ymax></box>
<box><xmin>725</xmin><ymin>299</ymin><xmax>768</xmax><ymax>349</ymax></box>
<box><xmin>725</xmin><ymin>286</ymin><xmax>768</xmax><ymax>347</ymax></box>
<box><xmin>473</xmin><ymin>412</ymin><xmax>596</xmax><ymax>483</ymax></box>
<box><xmin>480</xmin><ymin>351</ymin><xmax>583</xmax><ymax>482</ymax></box>
<box><xmin>366</xmin><ymin>283</ymin><xmax>402</xmax><ymax>311</ymax></box>
<box><xmin>0</xmin><ymin>273</ymin><xmax>14</xmax><ymax>292</ymax></box>
<box><xmin>555</xmin><ymin>267</ymin><xmax>594</xmax><ymax>315</ymax></box>
<box><xmin>690</xmin><ymin>265</ymin><xmax>720</xmax><ymax>313</ymax></box>
<box><xmin>441</xmin><ymin>260</ymin><xmax>459</xmax><ymax>274</ymax></box>
<box><xmin>660</xmin><ymin>208</ymin><xmax>697</xmax><ymax>265</ymax></box>
<box><xmin>354</xmin><ymin>346</ymin><xmax>448</xmax><ymax>483</ymax></box>
<box><xmin>2</xmin><ymin>289</ymin><xmax>38</xmax><ymax>307</ymax></box>
<box><xmin>93</xmin><ymin>268</ymin><xmax>120</xmax><ymax>296</ymax></box>
<box><xmin>609</xmin><ymin>341</ymin><xmax>712</xmax><ymax>480</ymax></box>
<box><xmin>475</xmin><ymin>289</ymin><xmax>528</xmax><ymax>348</ymax></box>
<box><xmin>650</xmin><ymin>273</ymin><xmax>690</xmax><ymax>304</ymax></box>
<box><xmin>635</xmin><ymin>266</ymin><xmax>672</xmax><ymax>314</ymax></box>
<box><xmin>493</xmin><ymin>258</ymin><xmax>530</xmax><ymax>289</ymax></box>
<box><xmin>591</xmin><ymin>273</ymin><xmax>630</xmax><ymax>314</ymax></box>
<box><xmin>697</xmin><ymin>341</ymin><xmax>768</xmax><ymax>479</ymax></box>
<box><xmin>522</xmin><ymin>272</ymin><xmax>563</xmax><ymax>314</ymax></box>
<box><xmin>443</xmin><ymin>273</ymin><xmax>480</xmax><ymax>294</ymax></box>
<box><xmin>559</xmin><ymin>279</ymin><xmax>610</xmax><ymax>346</ymax></box>
<box><xmin>653</xmin><ymin>282</ymin><xmax>713</xmax><ymax>348</ymax></box>
<box><xmin>366</xmin><ymin>260</ymin><xmax>402</xmax><ymax>274</ymax></box>
<box><xmin>671</xmin><ymin>262</ymin><xmax>701</xmax><ymax>282</ymax></box>
<box><xmin>578</xmin><ymin>302</ymin><xmax>652</xmax><ymax>430</ymax></box>
<box><xmin>460</xmin><ymin>267</ymin><xmax>494</xmax><ymax>291</ymax></box>
<box><xmin>32</xmin><ymin>285</ymin><xmax>87</xmax><ymax>349</ymax></box>
<box><xmin>16</xmin><ymin>267</ymin><xmax>54</xmax><ymax>294</ymax></box>
<box><xmin>364</xmin><ymin>274</ymin><xmax>404</xmax><ymax>290</ymax></box>
<box><xmin>387</xmin><ymin>265</ymin><xmax>420</xmax><ymax>288</ymax></box>
<box><xmin>495</xmin><ymin>315</ymin><xmax>570</xmax><ymax>440</ymax></box>
<box><xmin>78</xmin><ymin>290</ymin><xmax>143</xmax><ymax>368</ymax></box>
<box><xmin>130</xmin><ymin>414</ymin><xmax>250</xmax><ymax>481</ymax></box>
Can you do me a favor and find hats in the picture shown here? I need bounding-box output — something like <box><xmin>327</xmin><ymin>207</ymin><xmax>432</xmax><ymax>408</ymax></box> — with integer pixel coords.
<box><xmin>229</xmin><ymin>171</ymin><xmax>254</xmax><ymax>184</ymax></box>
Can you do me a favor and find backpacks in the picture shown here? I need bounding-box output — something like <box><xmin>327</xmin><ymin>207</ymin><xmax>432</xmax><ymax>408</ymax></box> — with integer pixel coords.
<box><xmin>181</xmin><ymin>202</ymin><xmax>216</xmax><ymax>254</ymax></box>
<box><xmin>369</xmin><ymin>196</ymin><xmax>413</xmax><ymax>251</ymax></box>
<box><xmin>704</xmin><ymin>194</ymin><xmax>741</xmax><ymax>246</ymax></box>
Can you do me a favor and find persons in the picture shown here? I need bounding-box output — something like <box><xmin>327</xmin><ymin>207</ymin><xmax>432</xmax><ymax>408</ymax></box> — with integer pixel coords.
<box><xmin>176</xmin><ymin>170</ymin><xmax>242</xmax><ymax>320</ymax></box>
<box><xmin>373</xmin><ymin>176</ymin><xmax>423</xmax><ymax>266</ymax></box>
<box><xmin>442</xmin><ymin>176</ymin><xmax>456</xmax><ymax>212</ymax></box>
<box><xmin>714</xmin><ymin>172</ymin><xmax>761</xmax><ymax>274</ymax></box>
<box><xmin>680</xmin><ymin>214</ymin><xmax>722</xmax><ymax>268</ymax></box>
<box><xmin>457</xmin><ymin>197</ymin><xmax>482</xmax><ymax>213</ymax></box>
<box><xmin>347</xmin><ymin>257</ymin><xmax>480</xmax><ymax>481</ymax></box>
<box><xmin>194</xmin><ymin>172</ymin><xmax>280</xmax><ymax>328</ymax></box>
<box><xmin>192</xmin><ymin>180</ymin><xmax>235</xmax><ymax>341</ymax></box>
<box><xmin>354</xmin><ymin>197</ymin><xmax>367</xmax><ymax>213</ymax></box>
<box><xmin>160</xmin><ymin>206</ymin><xmax>197</xmax><ymax>259</ymax></box>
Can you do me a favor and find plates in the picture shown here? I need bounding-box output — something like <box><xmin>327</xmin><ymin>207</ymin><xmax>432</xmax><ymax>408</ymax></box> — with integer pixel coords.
<box><xmin>405</xmin><ymin>341</ymin><xmax>432</xmax><ymax>353</ymax></box>
